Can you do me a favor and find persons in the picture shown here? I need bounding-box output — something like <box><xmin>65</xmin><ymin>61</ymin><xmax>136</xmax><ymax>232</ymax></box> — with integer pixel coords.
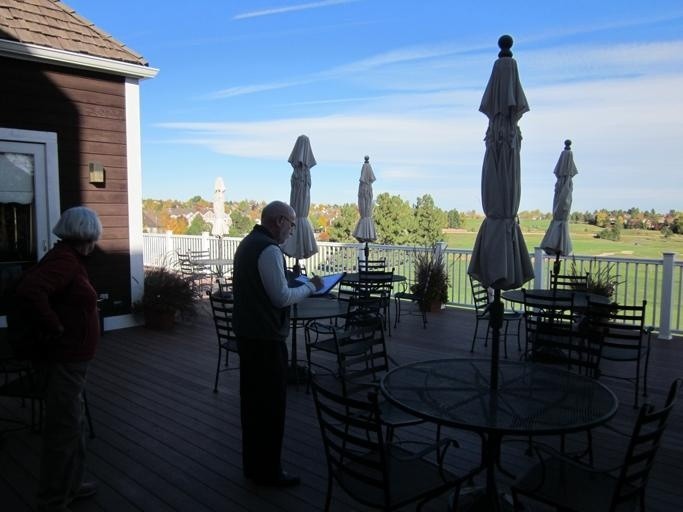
<box><xmin>8</xmin><ymin>206</ymin><xmax>101</xmax><ymax>512</ymax></box>
<box><xmin>231</xmin><ymin>200</ymin><xmax>325</xmax><ymax>489</ymax></box>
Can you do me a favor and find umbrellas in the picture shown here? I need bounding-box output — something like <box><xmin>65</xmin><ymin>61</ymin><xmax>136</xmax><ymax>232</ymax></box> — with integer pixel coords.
<box><xmin>467</xmin><ymin>34</ymin><xmax>529</xmax><ymax>415</ymax></box>
<box><xmin>279</xmin><ymin>135</ymin><xmax>318</xmax><ymax>367</ymax></box>
<box><xmin>539</xmin><ymin>140</ymin><xmax>578</xmax><ymax>324</ymax></box>
<box><xmin>352</xmin><ymin>156</ymin><xmax>377</xmax><ymax>290</ymax></box>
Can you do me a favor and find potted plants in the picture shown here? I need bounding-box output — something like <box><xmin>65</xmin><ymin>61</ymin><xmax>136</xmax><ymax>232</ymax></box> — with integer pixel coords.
<box><xmin>127</xmin><ymin>269</ymin><xmax>198</xmax><ymax>333</ymax></box>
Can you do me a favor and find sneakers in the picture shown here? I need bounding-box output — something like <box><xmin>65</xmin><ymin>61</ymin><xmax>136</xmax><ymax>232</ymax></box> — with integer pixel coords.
<box><xmin>74</xmin><ymin>480</ymin><xmax>97</xmax><ymax>496</ymax></box>
<box><xmin>255</xmin><ymin>468</ymin><xmax>301</xmax><ymax>488</ymax></box>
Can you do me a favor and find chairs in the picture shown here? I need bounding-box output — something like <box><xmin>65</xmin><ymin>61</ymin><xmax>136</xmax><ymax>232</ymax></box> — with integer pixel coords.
<box><xmin>1</xmin><ymin>358</ymin><xmax>97</xmax><ymax>441</ymax></box>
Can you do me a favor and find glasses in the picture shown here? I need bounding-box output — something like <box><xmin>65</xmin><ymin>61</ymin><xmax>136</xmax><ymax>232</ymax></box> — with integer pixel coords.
<box><xmin>279</xmin><ymin>214</ymin><xmax>297</xmax><ymax>231</ymax></box>
